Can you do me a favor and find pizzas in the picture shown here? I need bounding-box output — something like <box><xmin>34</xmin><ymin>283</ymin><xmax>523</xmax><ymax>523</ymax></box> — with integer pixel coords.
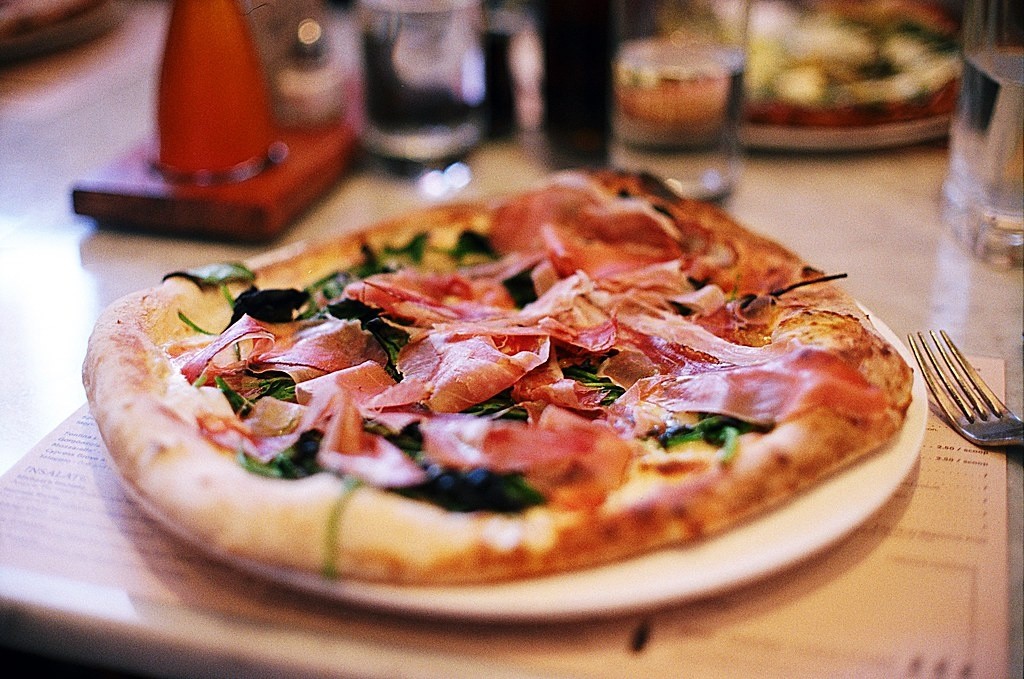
<box><xmin>85</xmin><ymin>165</ymin><xmax>910</xmax><ymax>581</ymax></box>
<box><xmin>732</xmin><ymin>4</ymin><xmax>964</xmax><ymax>128</ymax></box>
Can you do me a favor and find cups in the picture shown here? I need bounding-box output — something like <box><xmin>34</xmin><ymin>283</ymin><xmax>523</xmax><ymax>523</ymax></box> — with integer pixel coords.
<box><xmin>929</xmin><ymin>0</ymin><xmax>1024</xmax><ymax>419</ymax></box>
<box><xmin>611</xmin><ymin>0</ymin><xmax>745</xmax><ymax>207</ymax></box>
<box><xmin>356</xmin><ymin>0</ymin><xmax>487</xmax><ymax>175</ymax></box>
<box><xmin>157</xmin><ymin>0</ymin><xmax>273</xmax><ymax>185</ymax></box>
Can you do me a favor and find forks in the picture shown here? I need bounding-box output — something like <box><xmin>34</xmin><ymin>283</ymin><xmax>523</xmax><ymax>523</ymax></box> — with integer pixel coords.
<box><xmin>908</xmin><ymin>330</ymin><xmax>1024</xmax><ymax>447</ymax></box>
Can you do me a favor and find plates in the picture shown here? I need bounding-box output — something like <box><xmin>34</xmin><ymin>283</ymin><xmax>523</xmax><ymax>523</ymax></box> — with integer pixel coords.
<box><xmin>122</xmin><ymin>298</ymin><xmax>928</xmax><ymax>619</ymax></box>
<box><xmin>740</xmin><ymin>115</ymin><xmax>949</xmax><ymax>149</ymax></box>
